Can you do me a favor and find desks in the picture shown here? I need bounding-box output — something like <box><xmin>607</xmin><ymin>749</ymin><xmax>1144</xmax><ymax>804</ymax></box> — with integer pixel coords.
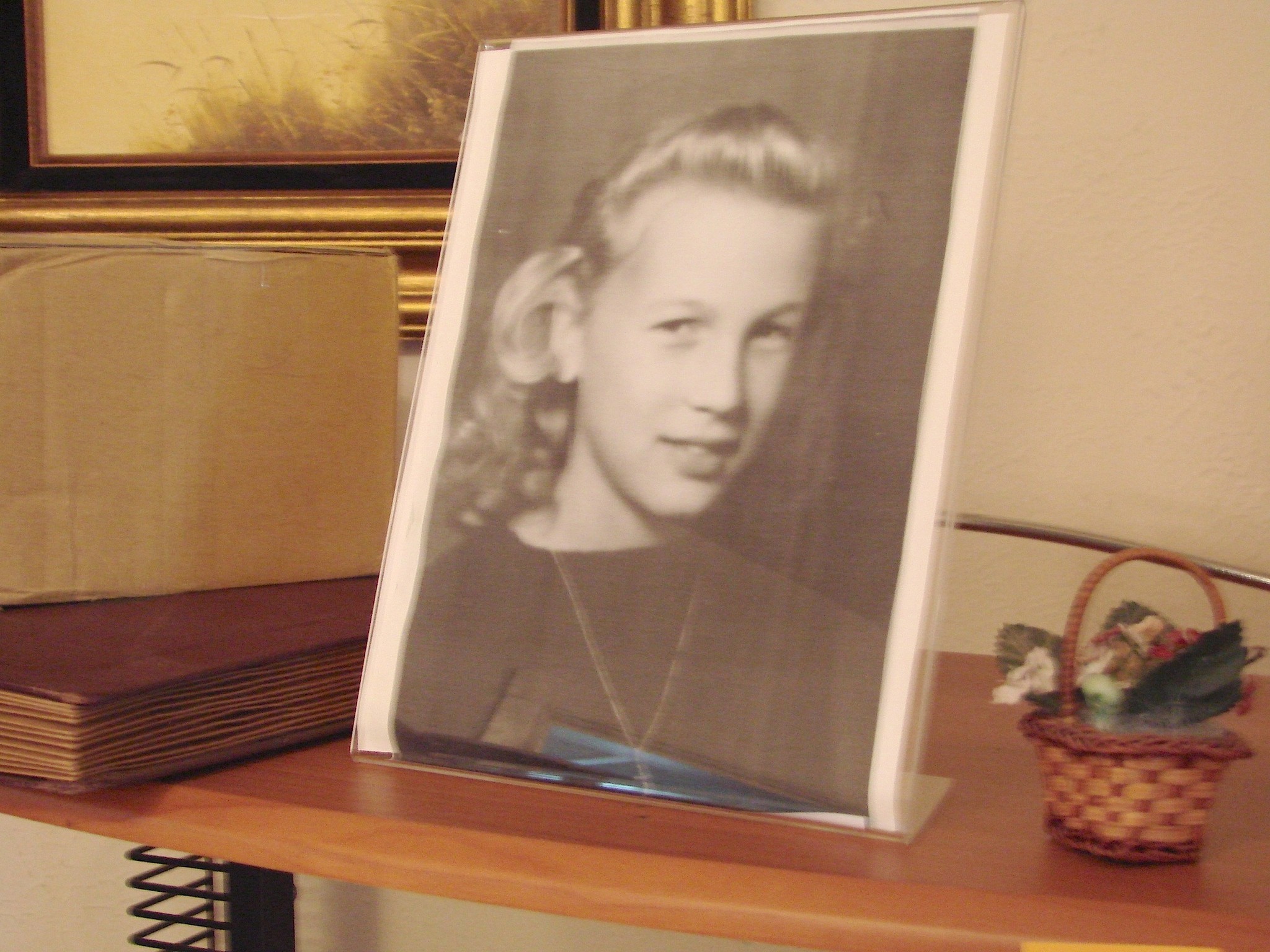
<box><xmin>0</xmin><ymin>652</ymin><xmax>1270</xmax><ymax>952</ymax></box>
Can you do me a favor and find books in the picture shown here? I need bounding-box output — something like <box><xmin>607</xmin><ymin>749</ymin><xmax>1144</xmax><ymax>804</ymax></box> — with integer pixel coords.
<box><xmin>0</xmin><ymin>572</ymin><xmax>381</xmax><ymax>792</ymax></box>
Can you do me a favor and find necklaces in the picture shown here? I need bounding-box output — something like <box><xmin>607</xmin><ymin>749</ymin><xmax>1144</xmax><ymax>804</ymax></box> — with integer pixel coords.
<box><xmin>541</xmin><ymin>530</ymin><xmax>704</xmax><ymax>792</ymax></box>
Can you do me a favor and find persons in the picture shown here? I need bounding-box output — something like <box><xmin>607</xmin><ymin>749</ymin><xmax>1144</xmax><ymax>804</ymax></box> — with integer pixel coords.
<box><xmin>399</xmin><ymin>100</ymin><xmax>892</xmax><ymax>817</ymax></box>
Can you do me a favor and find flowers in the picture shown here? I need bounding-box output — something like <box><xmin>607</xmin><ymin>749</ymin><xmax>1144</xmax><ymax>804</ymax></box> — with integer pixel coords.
<box><xmin>990</xmin><ymin>605</ymin><xmax>1266</xmax><ymax>728</ymax></box>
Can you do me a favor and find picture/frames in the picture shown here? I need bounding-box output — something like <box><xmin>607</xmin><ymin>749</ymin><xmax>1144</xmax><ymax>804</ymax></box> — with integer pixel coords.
<box><xmin>0</xmin><ymin>0</ymin><xmax>751</xmax><ymax>347</ymax></box>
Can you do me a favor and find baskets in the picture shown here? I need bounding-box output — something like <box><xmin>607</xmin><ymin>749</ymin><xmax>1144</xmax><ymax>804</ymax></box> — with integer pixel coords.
<box><xmin>1020</xmin><ymin>549</ymin><xmax>1257</xmax><ymax>860</ymax></box>
